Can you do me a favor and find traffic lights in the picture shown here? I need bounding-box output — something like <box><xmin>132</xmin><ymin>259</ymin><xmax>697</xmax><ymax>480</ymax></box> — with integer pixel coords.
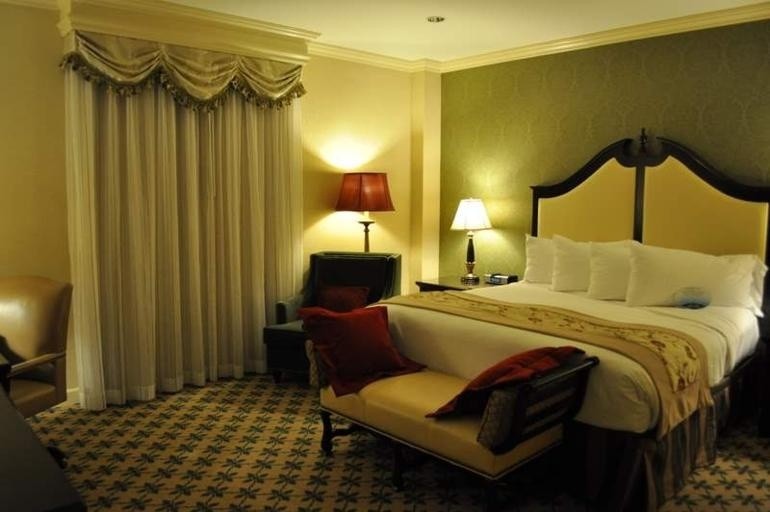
<box><xmin>626</xmin><ymin>240</ymin><xmax>768</xmax><ymax>317</ymax></box>
<box><xmin>523</xmin><ymin>233</ymin><xmax>553</xmax><ymax>283</ymax></box>
<box><xmin>549</xmin><ymin>234</ymin><xmax>590</xmax><ymax>292</ymax></box>
<box><xmin>425</xmin><ymin>346</ymin><xmax>585</xmax><ymax>419</ymax></box>
<box><xmin>586</xmin><ymin>241</ymin><xmax>629</xmax><ymax>300</ymax></box>
<box><xmin>316</xmin><ymin>281</ymin><xmax>370</xmax><ymax>312</ymax></box>
<box><xmin>296</xmin><ymin>306</ymin><xmax>427</xmax><ymax>397</ymax></box>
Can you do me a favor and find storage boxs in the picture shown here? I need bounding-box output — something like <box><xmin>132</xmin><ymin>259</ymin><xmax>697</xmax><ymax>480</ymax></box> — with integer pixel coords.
<box><xmin>305</xmin><ymin>321</ymin><xmax>599</xmax><ymax>488</ymax></box>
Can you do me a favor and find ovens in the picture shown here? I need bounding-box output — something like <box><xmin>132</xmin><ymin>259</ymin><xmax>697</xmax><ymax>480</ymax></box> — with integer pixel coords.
<box><xmin>366</xmin><ymin>126</ymin><xmax>770</xmax><ymax>512</ymax></box>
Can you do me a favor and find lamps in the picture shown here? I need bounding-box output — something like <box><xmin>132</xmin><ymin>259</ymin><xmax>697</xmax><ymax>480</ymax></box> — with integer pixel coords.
<box><xmin>263</xmin><ymin>251</ymin><xmax>401</xmax><ymax>383</ymax></box>
<box><xmin>0</xmin><ymin>276</ymin><xmax>74</xmax><ymax>469</ymax></box>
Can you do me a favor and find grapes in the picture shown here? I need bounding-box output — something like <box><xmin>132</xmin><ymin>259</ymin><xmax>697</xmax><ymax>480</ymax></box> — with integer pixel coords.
<box><xmin>416</xmin><ymin>277</ymin><xmax>501</xmax><ymax>292</ymax></box>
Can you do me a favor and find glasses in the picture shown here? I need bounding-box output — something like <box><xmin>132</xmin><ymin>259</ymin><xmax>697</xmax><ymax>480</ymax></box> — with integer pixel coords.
<box><xmin>450</xmin><ymin>198</ymin><xmax>492</xmax><ymax>282</ymax></box>
<box><xmin>335</xmin><ymin>173</ymin><xmax>395</xmax><ymax>253</ymax></box>
